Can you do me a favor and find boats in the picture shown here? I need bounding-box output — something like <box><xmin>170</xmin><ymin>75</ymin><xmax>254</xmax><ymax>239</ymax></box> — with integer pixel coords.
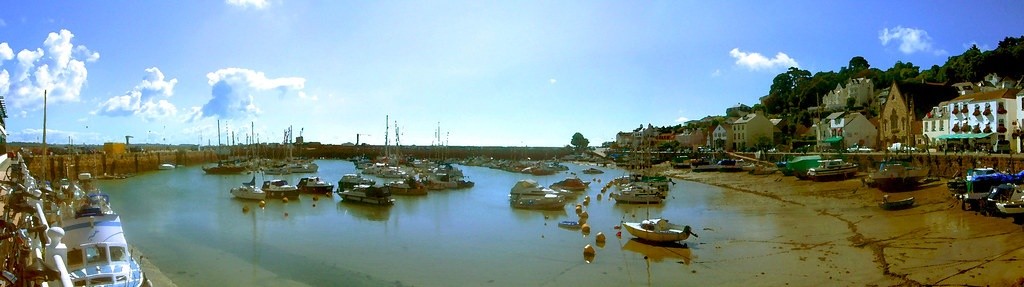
<box><xmin>879</xmin><ymin>196</ymin><xmax>914</xmax><ymax>210</ymax></box>
<box><xmin>987</xmin><ymin>184</ymin><xmax>1024</xmax><ymax>218</ymax></box>
<box><xmin>548</xmin><ymin>178</ymin><xmax>589</xmax><ymax>191</ymax></box>
<box><xmin>37</xmin><ymin>172</ymin><xmax>145</xmax><ymax>287</ymax></box>
<box><xmin>948</xmin><ymin>168</ymin><xmax>1002</xmax><ymax>206</ymax></box>
<box><xmin>864</xmin><ymin>165</ymin><xmax>931</xmax><ymax>187</ymax></box>
<box><xmin>510</xmin><ymin>180</ymin><xmax>568</xmax><ymax>209</ymax></box>
<box><xmin>158</xmin><ymin>164</ymin><xmax>175</xmax><ymax>170</ymax></box>
<box><xmin>201</xmin><ymin>118</ymin><xmax>335</xmax><ymax>201</ymax></box>
<box><xmin>559</xmin><ymin>221</ymin><xmax>579</xmax><ymax>227</ymax></box>
<box><xmin>458</xmin><ymin>143</ymin><xmax>569</xmax><ymax>176</ymax></box>
<box><xmin>610</xmin><ymin>172</ymin><xmax>669</xmax><ymax>204</ymax></box>
<box><xmin>806</xmin><ymin>160</ymin><xmax>859</xmax><ymax>179</ymax></box>
<box><xmin>337</xmin><ymin>114</ymin><xmax>476</xmax><ymax>206</ymax></box>
<box><xmin>622</xmin><ymin>219</ymin><xmax>692</xmax><ymax>242</ymax></box>
<box><xmin>583</xmin><ymin>168</ymin><xmax>604</xmax><ymax>174</ymax></box>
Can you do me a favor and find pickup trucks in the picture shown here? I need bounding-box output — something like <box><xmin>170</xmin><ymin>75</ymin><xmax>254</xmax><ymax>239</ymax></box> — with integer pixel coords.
<box><xmin>887</xmin><ymin>143</ymin><xmax>915</xmax><ymax>152</ymax></box>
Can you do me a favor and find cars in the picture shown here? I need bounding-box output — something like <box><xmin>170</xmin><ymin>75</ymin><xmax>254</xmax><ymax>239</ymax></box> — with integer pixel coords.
<box><xmin>848</xmin><ymin>144</ymin><xmax>875</xmax><ymax>152</ymax></box>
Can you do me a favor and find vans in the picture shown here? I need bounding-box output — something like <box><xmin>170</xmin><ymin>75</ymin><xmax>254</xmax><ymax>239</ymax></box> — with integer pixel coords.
<box><xmin>993</xmin><ymin>139</ymin><xmax>1010</xmax><ymax>153</ymax></box>
